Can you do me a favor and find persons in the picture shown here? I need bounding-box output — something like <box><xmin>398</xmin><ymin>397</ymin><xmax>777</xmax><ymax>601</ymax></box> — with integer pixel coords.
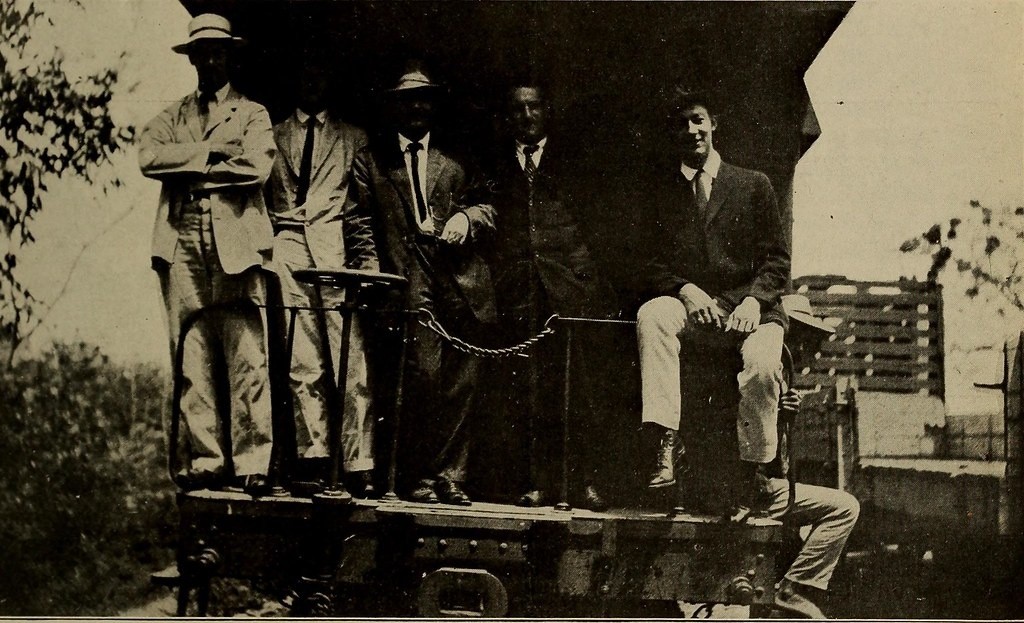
<box><xmin>342</xmin><ymin>59</ymin><xmax>503</xmax><ymax>506</ymax></box>
<box><xmin>474</xmin><ymin>76</ymin><xmax>625</xmax><ymax>511</ymax></box>
<box><xmin>695</xmin><ymin>293</ymin><xmax>861</xmax><ymax>620</ymax></box>
<box><xmin>260</xmin><ymin>58</ymin><xmax>394</xmax><ymax>497</ymax></box>
<box><xmin>630</xmin><ymin>82</ymin><xmax>790</xmax><ymax>498</ymax></box>
<box><xmin>135</xmin><ymin>13</ymin><xmax>278</xmax><ymax>497</ymax></box>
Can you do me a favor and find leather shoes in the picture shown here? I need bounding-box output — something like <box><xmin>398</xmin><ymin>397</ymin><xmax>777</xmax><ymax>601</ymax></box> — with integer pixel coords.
<box><xmin>513</xmin><ymin>490</ymin><xmax>549</xmax><ymax>507</ymax></box>
<box><xmin>287</xmin><ymin>467</ymin><xmax>328</xmax><ymax>497</ymax></box>
<box><xmin>394</xmin><ymin>478</ymin><xmax>472</xmax><ymax>505</ymax></box>
<box><xmin>575</xmin><ymin>483</ymin><xmax>609</xmax><ymax>511</ymax></box>
<box><xmin>740</xmin><ymin>462</ymin><xmax>773</xmax><ymax>506</ymax></box>
<box><xmin>648</xmin><ymin>429</ymin><xmax>687</xmax><ymax>489</ymax></box>
<box><xmin>345</xmin><ymin>471</ymin><xmax>386</xmax><ymax>498</ymax></box>
<box><xmin>242</xmin><ymin>474</ymin><xmax>274</xmax><ymax>498</ymax></box>
<box><xmin>173</xmin><ymin>469</ymin><xmax>225</xmax><ymax>492</ymax></box>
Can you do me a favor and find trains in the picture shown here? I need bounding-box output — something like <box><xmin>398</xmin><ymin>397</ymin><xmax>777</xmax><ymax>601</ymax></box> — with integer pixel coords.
<box><xmin>167</xmin><ymin>0</ymin><xmax>855</xmax><ymax>622</ymax></box>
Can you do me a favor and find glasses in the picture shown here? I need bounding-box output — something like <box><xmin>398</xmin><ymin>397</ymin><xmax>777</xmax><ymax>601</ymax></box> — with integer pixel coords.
<box><xmin>512</xmin><ymin>102</ymin><xmax>543</xmax><ymax>113</ymax></box>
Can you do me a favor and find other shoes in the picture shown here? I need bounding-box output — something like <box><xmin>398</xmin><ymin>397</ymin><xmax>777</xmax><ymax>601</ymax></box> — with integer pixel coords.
<box><xmin>773</xmin><ymin>594</ymin><xmax>827</xmax><ymax>619</ymax></box>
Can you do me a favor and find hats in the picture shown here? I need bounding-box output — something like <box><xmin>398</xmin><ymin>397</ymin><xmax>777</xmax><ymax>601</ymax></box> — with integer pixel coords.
<box><xmin>386</xmin><ymin>60</ymin><xmax>440</xmax><ymax>94</ymax></box>
<box><xmin>171</xmin><ymin>13</ymin><xmax>244</xmax><ymax>55</ymax></box>
<box><xmin>781</xmin><ymin>294</ymin><xmax>836</xmax><ymax>333</ymax></box>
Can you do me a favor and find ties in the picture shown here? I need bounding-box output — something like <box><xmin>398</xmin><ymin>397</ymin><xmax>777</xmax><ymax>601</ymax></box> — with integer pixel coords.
<box><xmin>524</xmin><ymin>145</ymin><xmax>540</xmax><ymax>196</ymax></box>
<box><xmin>294</xmin><ymin>117</ymin><xmax>316</xmax><ymax>208</ymax></box>
<box><xmin>407</xmin><ymin>143</ymin><xmax>427</xmax><ymax>223</ymax></box>
<box><xmin>198</xmin><ymin>93</ymin><xmax>217</xmax><ymax>136</ymax></box>
<box><xmin>694</xmin><ymin>171</ymin><xmax>709</xmax><ymax>224</ymax></box>
